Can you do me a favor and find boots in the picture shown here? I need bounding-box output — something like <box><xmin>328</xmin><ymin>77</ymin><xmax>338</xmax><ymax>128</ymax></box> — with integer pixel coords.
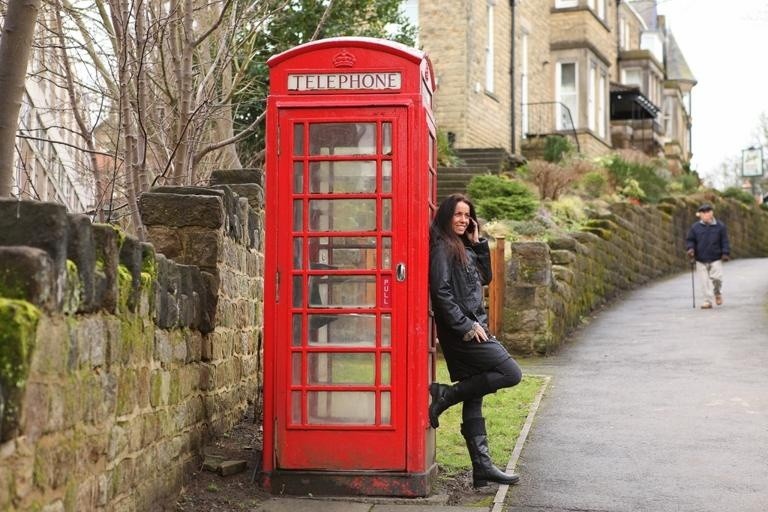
<box><xmin>430</xmin><ymin>374</ymin><xmax>496</xmax><ymax>429</ymax></box>
<box><xmin>460</xmin><ymin>418</ymin><xmax>520</xmax><ymax>486</ymax></box>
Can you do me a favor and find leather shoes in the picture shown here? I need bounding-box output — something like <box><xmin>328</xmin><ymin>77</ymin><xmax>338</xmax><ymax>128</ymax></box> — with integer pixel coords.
<box><xmin>702</xmin><ymin>296</ymin><xmax>722</xmax><ymax>308</ymax></box>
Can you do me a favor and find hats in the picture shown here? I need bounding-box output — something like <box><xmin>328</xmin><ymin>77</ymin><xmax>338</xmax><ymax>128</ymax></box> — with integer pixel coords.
<box><xmin>699</xmin><ymin>204</ymin><xmax>712</xmax><ymax>212</ymax></box>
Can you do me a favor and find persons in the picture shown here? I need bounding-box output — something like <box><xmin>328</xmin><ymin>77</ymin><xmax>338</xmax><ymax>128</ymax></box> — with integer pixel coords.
<box><xmin>686</xmin><ymin>205</ymin><xmax>729</xmax><ymax>308</ymax></box>
<box><xmin>429</xmin><ymin>193</ymin><xmax>520</xmax><ymax>487</ymax></box>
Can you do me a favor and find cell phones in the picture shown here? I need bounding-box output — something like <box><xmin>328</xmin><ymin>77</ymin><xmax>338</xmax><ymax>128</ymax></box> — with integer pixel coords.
<box><xmin>466</xmin><ymin>219</ymin><xmax>475</xmax><ymax>233</ymax></box>
